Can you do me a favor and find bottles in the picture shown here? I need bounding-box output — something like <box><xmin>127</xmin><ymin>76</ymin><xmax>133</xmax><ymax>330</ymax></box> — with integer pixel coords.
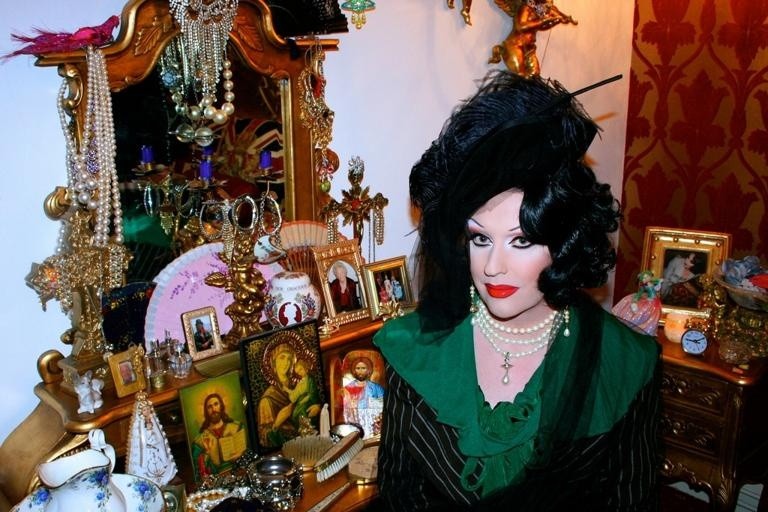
<box><xmin>147</xmin><ymin>329</ymin><xmax>194</xmax><ymax>386</ymax></box>
<box><xmin>263</xmin><ymin>271</ymin><xmax>321</xmax><ymax>333</ymax></box>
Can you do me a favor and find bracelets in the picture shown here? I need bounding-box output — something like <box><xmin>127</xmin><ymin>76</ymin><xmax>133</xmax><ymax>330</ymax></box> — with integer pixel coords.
<box><xmin>198</xmin><ymin>195</ymin><xmax>282</xmax><ymax>240</ymax></box>
<box><xmin>186</xmin><ymin>488</ymin><xmax>229</xmax><ymax>512</ymax></box>
<box><xmin>375</xmin><ymin>212</ymin><xmax>384</xmax><ymax>245</ymax></box>
<box><xmin>143</xmin><ymin>178</ymin><xmax>196</xmax><ymax>218</ymax></box>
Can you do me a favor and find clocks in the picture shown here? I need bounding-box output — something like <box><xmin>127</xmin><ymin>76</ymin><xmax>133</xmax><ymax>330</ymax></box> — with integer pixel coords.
<box><xmin>679</xmin><ymin>324</ymin><xmax>710</xmax><ymax>356</ymax></box>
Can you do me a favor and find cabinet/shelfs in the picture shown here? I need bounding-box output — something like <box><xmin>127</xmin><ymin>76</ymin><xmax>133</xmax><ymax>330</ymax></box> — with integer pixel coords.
<box><xmin>645</xmin><ymin>330</ymin><xmax>767</xmax><ymax>512</ymax></box>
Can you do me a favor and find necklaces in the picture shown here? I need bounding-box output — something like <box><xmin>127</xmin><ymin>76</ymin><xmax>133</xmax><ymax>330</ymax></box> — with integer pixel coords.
<box><xmin>159</xmin><ymin>0</ymin><xmax>239</xmax><ymax>147</ymax></box>
<box><xmin>468</xmin><ymin>282</ymin><xmax>570</xmax><ymax>384</ymax></box>
<box><xmin>57</xmin><ymin>45</ymin><xmax>126</xmax><ymax>249</ymax></box>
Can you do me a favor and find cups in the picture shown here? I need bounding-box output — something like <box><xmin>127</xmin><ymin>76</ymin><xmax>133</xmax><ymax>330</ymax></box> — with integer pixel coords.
<box><xmin>664</xmin><ymin>312</ymin><xmax>691</xmax><ymax>343</ymax></box>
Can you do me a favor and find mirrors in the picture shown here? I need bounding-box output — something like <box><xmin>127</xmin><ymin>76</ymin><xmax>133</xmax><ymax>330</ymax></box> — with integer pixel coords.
<box><xmin>102</xmin><ymin>20</ymin><xmax>285</xmax><ymax>285</ymax></box>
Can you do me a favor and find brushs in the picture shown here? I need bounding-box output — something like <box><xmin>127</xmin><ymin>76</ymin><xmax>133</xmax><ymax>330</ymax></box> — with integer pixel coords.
<box><xmin>313</xmin><ymin>431</ymin><xmax>365</xmax><ymax>483</ymax></box>
<box><xmin>281</xmin><ymin>434</ymin><xmax>333</xmax><ymax>472</ymax></box>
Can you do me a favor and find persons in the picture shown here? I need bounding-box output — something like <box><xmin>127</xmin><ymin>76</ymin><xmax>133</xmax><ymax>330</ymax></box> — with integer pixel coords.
<box><xmin>120</xmin><ymin>364</ymin><xmax>132</xmax><ymax>384</ymax></box>
<box><xmin>191</xmin><ymin>393</ymin><xmax>251</xmax><ymax>481</ymax></box>
<box><xmin>661</xmin><ymin>251</ymin><xmax>700</xmax><ymax>305</ymax></box>
<box><xmin>611</xmin><ymin>271</ymin><xmax>663</xmax><ymax>338</ymax></box>
<box><xmin>500</xmin><ymin>0</ymin><xmax>571</xmax><ymax>79</ymax></box>
<box><xmin>125</xmin><ymin>399</ymin><xmax>179</xmax><ymax>487</ymax></box>
<box><xmin>343</xmin><ymin>357</ymin><xmax>386</xmax><ymax>440</ymax></box>
<box><xmin>330</xmin><ymin>262</ymin><xmax>361</xmax><ymax>313</ymax></box>
<box><xmin>376</xmin><ymin>70</ymin><xmax>666</xmax><ymax>512</ymax></box>
<box><xmin>282</xmin><ymin>358</ymin><xmax>320</xmax><ymax>437</ymax></box>
<box><xmin>194</xmin><ymin>319</ymin><xmax>213</xmax><ymax>352</ymax></box>
<box><xmin>376</xmin><ymin>274</ymin><xmax>403</xmax><ymax>302</ymax></box>
<box><xmin>257</xmin><ymin>344</ymin><xmax>322</xmax><ymax>447</ymax></box>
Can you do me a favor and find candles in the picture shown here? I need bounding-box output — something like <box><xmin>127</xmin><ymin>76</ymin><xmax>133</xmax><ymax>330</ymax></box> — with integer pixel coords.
<box><xmin>260</xmin><ymin>149</ymin><xmax>271</xmax><ymax>167</ymax></box>
<box><xmin>204</xmin><ymin>146</ymin><xmax>212</xmax><ymax>156</ymax></box>
<box><xmin>140</xmin><ymin>145</ymin><xmax>152</xmax><ymax>162</ymax></box>
<box><xmin>200</xmin><ymin>161</ymin><xmax>211</xmax><ymax>176</ymax></box>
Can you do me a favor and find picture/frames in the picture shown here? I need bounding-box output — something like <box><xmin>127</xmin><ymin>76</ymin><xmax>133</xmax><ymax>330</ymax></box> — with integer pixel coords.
<box><xmin>180</xmin><ymin>306</ymin><xmax>220</xmax><ymax>357</ymax></box>
<box><xmin>361</xmin><ymin>255</ymin><xmax>416</xmax><ymax>321</ymax></box>
<box><xmin>109</xmin><ymin>341</ymin><xmax>146</xmax><ymax>397</ymax></box>
<box><xmin>313</xmin><ymin>243</ymin><xmax>376</xmax><ymax>329</ymax></box>
<box><xmin>237</xmin><ymin>319</ymin><xmax>329</xmax><ymax>454</ymax></box>
<box><xmin>644</xmin><ymin>228</ymin><xmax>730</xmax><ymax>326</ymax></box>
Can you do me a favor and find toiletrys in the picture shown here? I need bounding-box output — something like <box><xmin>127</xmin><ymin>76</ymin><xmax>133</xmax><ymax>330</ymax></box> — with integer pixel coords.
<box><xmin>147</xmin><ymin>331</ymin><xmax>192</xmax><ymax>389</ymax></box>
